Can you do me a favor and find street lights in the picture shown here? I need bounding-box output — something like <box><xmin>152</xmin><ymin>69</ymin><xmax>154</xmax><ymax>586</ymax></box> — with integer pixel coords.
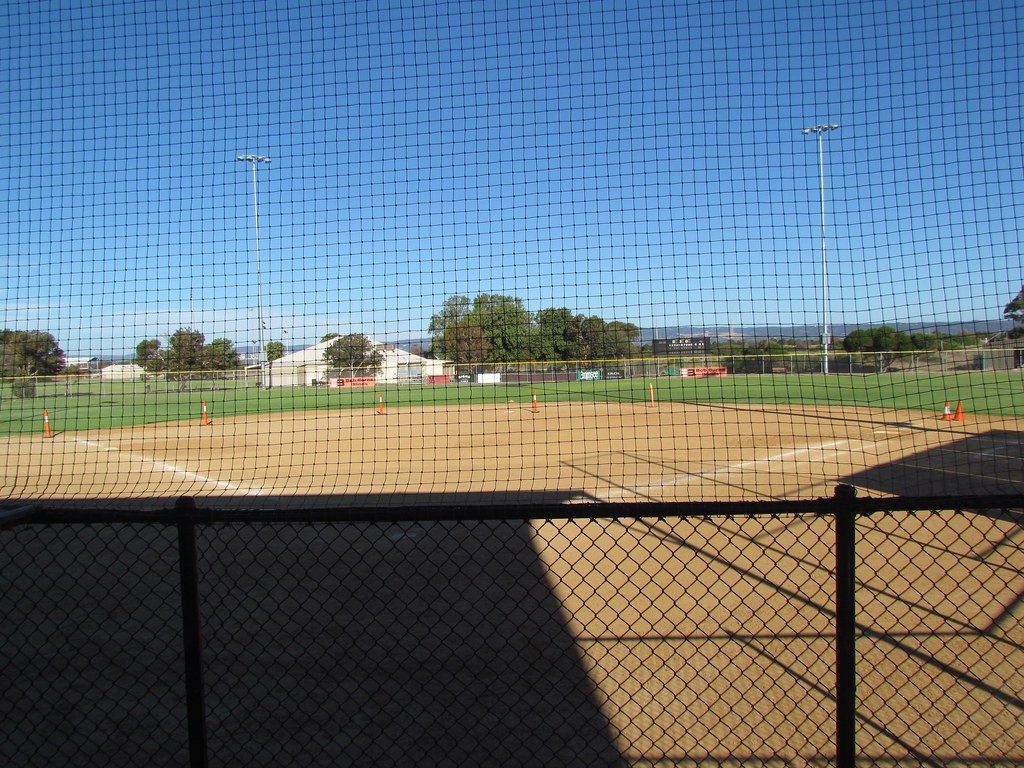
<box><xmin>234</xmin><ymin>154</ymin><xmax>273</xmax><ymax>393</ymax></box>
<box><xmin>801</xmin><ymin>124</ymin><xmax>840</xmax><ymax>376</ymax></box>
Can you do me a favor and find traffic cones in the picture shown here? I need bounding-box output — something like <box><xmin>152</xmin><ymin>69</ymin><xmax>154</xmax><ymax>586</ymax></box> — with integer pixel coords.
<box><xmin>952</xmin><ymin>400</ymin><xmax>964</xmax><ymax>421</ymax></box>
<box><xmin>941</xmin><ymin>400</ymin><xmax>954</xmax><ymax>421</ymax></box>
